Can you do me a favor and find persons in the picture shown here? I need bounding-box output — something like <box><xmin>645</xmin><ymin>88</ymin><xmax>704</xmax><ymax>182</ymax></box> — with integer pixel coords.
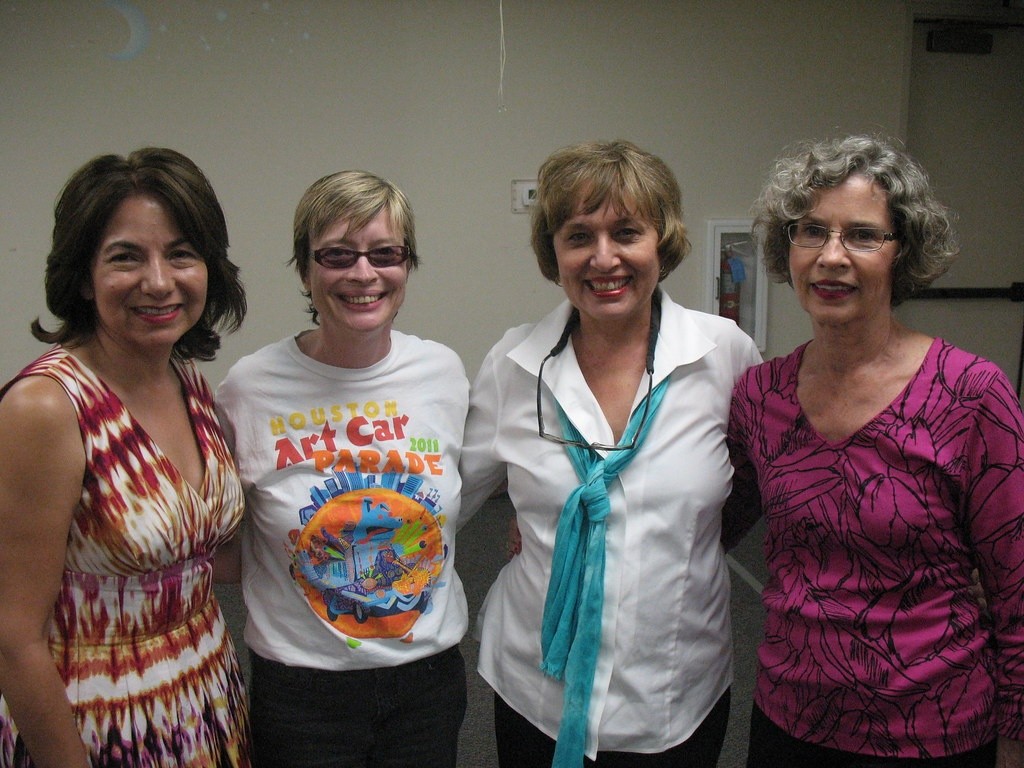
<box><xmin>456</xmin><ymin>143</ymin><xmax>764</xmax><ymax>768</ymax></box>
<box><xmin>0</xmin><ymin>148</ymin><xmax>250</xmax><ymax>768</ymax></box>
<box><xmin>505</xmin><ymin>132</ymin><xmax>1024</xmax><ymax>768</ymax></box>
<box><xmin>214</xmin><ymin>169</ymin><xmax>472</xmax><ymax>768</ymax></box>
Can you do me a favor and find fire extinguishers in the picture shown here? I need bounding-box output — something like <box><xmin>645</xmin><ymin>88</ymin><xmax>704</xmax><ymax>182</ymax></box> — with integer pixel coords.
<box><xmin>721</xmin><ymin>241</ymin><xmax>748</xmax><ymax>327</ymax></box>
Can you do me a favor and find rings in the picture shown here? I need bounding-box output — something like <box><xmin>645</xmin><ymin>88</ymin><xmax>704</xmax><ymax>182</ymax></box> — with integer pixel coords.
<box><xmin>506</xmin><ymin>542</ymin><xmax>509</xmax><ymax>549</ymax></box>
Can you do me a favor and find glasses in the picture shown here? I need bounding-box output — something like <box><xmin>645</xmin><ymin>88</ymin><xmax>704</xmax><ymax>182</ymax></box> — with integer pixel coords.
<box><xmin>536</xmin><ymin>353</ymin><xmax>652</xmax><ymax>451</ymax></box>
<box><xmin>305</xmin><ymin>246</ymin><xmax>411</xmax><ymax>269</ymax></box>
<box><xmin>783</xmin><ymin>220</ymin><xmax>902</xmax><ymax>252</ymax></box>
